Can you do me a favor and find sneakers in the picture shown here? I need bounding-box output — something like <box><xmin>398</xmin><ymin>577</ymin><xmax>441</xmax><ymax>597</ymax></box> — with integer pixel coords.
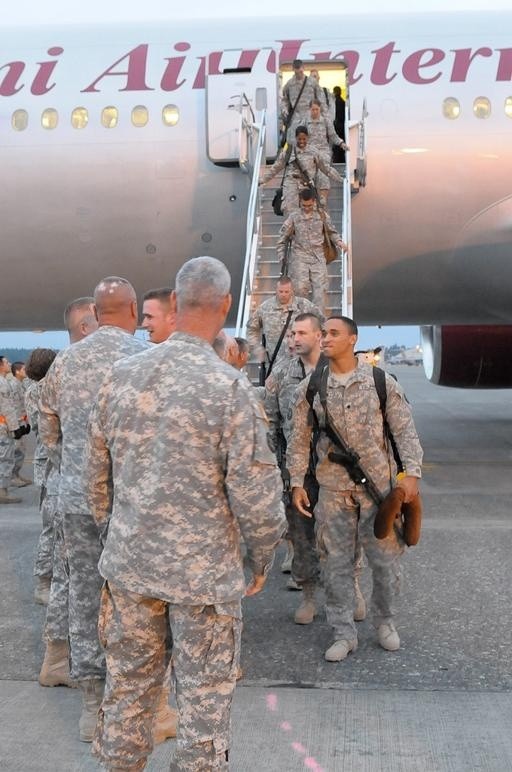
<box><xmin>9</xmin><ymin>465</ymin><xmax>34</xmax><ymax>487</ymax></box>
<box><xmin>323</xmin><ymin>636</ymin><xmax>358</xmax><ymax>663</ymax></box>
<box><xmin>285</xmin><ymin>574</ymin><xmax>304</xmax><ymax>591</ymax></box>
<box><xmin>376</xmin><ymin>622</ymin><xmax>400</xmax><ymax>652</ymax></box>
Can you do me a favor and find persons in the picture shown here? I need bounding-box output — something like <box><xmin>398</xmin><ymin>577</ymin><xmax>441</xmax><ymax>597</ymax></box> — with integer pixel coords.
<box><xmin>23</xmin><ymin>255</ymin><xmax>288</xmax><ymax>771</ymax></box>
<box><xmin>0</xmin><ymin>356</ymin><xmax>36</xmax><ymax>505</ymax></box>
<box><xmin>248</xmin><ymin>278</ymin><xmax>325</xmax><ymax>378</ymax></box>
<box><xmin>258</xmin><ymin>60</ymin><xmax>350</xmax><ymax>319</ymax></box>
<box><xmin>263</xmin><ymin>312</ymin><xmax>423</xmax><ymax>663</ymax></box>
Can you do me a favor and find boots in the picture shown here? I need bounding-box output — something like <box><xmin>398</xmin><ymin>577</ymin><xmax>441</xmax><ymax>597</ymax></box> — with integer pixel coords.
<box><xmin>0</xmin><ymin>487</ymin><xmax>22</xmax><ymax>504</ymax></box>
<box><xmin>77</xmin><ymin>677</ymin><xmax>106</xmax><ymax>744</ymax></box>
<box><xmin>33</xmin><ymin>576</ymin><xmax>53</xmax><ymax>609</ymax></box>
<box><xmin>154</xmin><ymin>686</ymin><xmax>179</xmax><ymax>746</ymax></box>
<box><xmin>280</xmin><ymin>538</ymin><xmax>295</xmax><ymax>574</ymax></box>
<box><xmin>293</xmin><ymin>582</ymin><xmax>317</xmax><ymax>626</ymax></box>
<box><xmin>351</xmin><ymin>575</ymin><xmax>366</xmax><ymax>622</ymax></box>
<box><xmin>37</xmin><ymin>639</ymin><xmax>77</xmax><ymax>692</ymax></box>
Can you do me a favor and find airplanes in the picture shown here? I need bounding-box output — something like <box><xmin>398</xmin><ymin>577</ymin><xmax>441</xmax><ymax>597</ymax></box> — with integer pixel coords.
<box><xmin>0</xmin><ymin>18</ymin><xmax>512</xmax><ymax>395</ymax></box>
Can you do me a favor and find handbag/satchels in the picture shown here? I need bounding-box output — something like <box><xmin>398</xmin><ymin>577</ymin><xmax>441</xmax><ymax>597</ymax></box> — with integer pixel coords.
<box><xmin>282</xmin><ymin>107</ymin><xmax>295</xmax><ymax>124</ymax></box>
<box><xmin>270</xmin><ymin>188</ymin><xmax>284</xmax><ymax>217</ymax></box>
<box><xmin>322</xmin><ymin>236</ymin><xmax>339</xmax><ymax>266</ymax></box>
